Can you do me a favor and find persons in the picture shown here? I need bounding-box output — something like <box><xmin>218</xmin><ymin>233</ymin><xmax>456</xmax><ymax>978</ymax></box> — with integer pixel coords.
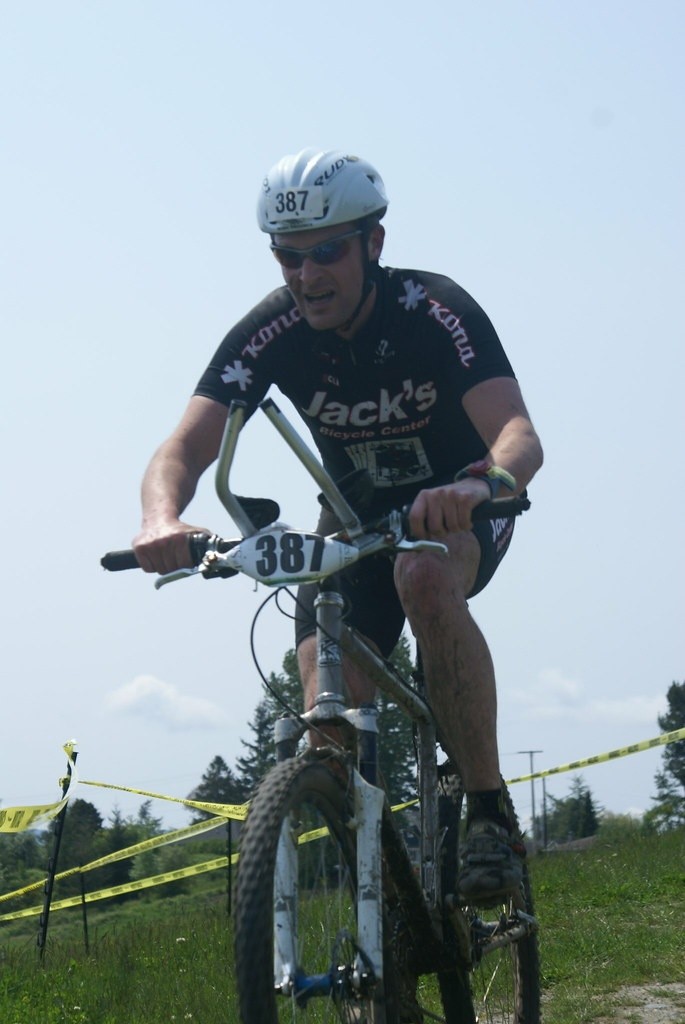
<box><xmin>132</xmin><ymin>151</ymin><xmax>542</xmax><ymax>1024</ymax></box>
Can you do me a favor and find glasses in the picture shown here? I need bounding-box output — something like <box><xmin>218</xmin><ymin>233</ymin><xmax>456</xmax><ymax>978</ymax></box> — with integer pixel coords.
<box><xmin>270</xmin><ymin>230</ymin><xmax>364</xmax><ymax>269</ymax></box>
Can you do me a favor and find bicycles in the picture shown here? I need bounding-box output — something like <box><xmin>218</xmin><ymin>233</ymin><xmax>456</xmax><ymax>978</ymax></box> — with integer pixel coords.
<box><xmin>98</xmin><ymin>399</ymin><xmax>544</xmax><ymax>1024</ymax></box>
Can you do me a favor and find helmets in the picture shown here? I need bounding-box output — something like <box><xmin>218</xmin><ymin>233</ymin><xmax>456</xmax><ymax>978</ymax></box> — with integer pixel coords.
<box><xmin>256</xmin><ymin>147</ymin><xmax>389</xmax><ymax>235</ymax></box>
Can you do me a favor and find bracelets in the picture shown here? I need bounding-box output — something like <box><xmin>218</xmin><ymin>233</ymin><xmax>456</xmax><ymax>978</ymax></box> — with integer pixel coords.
<box><xmin>456</xmin><ymin>459</ymin><xmax>517</xmax><ymax>498</ymax></box>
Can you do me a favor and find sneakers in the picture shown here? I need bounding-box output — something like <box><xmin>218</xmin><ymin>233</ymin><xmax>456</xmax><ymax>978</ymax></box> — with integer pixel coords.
<box><xmin>457</xmin><ymin>820</ymin><xmax>524</xmax><ymax>910</ymax></box>
